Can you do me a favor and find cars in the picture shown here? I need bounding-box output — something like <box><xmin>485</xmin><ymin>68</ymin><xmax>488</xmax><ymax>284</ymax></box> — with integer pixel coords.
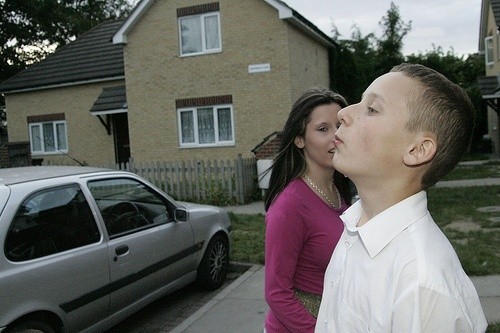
<box><xmin>0</xmin><ymin>166</ymin><xmax>232</xmax><ymax>333</ymax></box>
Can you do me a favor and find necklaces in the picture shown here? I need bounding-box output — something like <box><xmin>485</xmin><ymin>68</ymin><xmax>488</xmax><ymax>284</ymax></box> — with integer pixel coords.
<box><xmin>300</xmin><ymin>169</ymin><xmax>342</xmax><ymax>212</ymax></box>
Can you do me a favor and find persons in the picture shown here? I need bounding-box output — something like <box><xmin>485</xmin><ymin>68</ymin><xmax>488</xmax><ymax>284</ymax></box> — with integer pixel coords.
<box><xmin>313</xmin><ymin>64</ymin><xmax>490</xmax><ymax>333</ymax></box>
<box><xmin>264</xmin><ymin>88</ymin><xmax>357</xmax><ymax>333</ymax></box>
